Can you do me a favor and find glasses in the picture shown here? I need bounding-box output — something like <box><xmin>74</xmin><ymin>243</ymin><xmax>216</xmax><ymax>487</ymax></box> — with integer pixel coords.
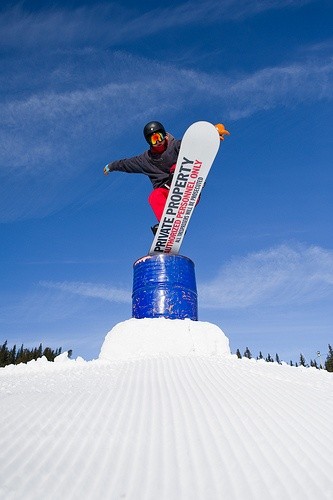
<box><xmin>147</xmin><ymin>132</ymin><xmax>165</xmax><ymax>145</ymax></box>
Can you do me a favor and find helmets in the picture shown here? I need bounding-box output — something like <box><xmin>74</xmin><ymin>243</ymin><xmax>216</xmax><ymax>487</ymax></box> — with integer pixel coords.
<box><xmin>144</xmin><ymin>121</ymin><xmax>165</xmax><ymax>141</ymax></box>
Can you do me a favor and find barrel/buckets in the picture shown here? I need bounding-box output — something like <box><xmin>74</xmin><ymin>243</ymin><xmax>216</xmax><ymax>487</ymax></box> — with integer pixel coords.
<box><xmin>131</xmin><ymin>254</ymin><xmax>198</xmax><ymax>322</ymax></box>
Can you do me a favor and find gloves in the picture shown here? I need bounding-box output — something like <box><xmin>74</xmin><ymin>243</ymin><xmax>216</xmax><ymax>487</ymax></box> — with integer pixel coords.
<box><xmin>104</xmin><ymin>164</ymin><xmax>111</xmax><ymax>175</ymax></box>
<box><xmin>215</xmin><ymin>124</ymin><xmax>230</xmax><ymax>141</ymax></box>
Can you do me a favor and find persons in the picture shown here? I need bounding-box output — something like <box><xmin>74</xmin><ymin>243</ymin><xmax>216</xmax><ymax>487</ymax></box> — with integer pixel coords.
<box><xmin>104</xmin><ymin>121</ymin><xmax>230</xmax><ymax>236</ymax></box>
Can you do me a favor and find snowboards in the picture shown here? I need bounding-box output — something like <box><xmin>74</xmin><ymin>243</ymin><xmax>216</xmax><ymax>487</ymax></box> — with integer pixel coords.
<box><xmin>149</xmin><ymin>120</ymin><xmax>220</xmax><ymax>255</ymax></box>
